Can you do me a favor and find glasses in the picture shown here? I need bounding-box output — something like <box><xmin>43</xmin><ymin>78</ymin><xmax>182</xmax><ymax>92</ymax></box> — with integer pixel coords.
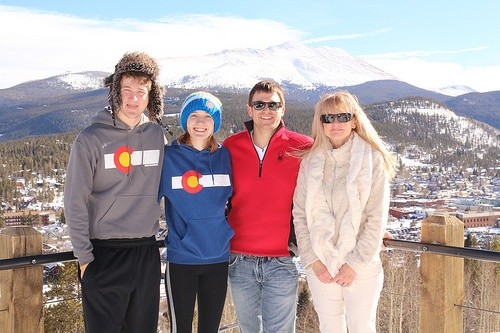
<box><xmin>320</xmin><ymin>113</ymin><xmax>354</xmax><ymax>123</ymax></box>
<box><xmin>250</xmin><ymin>101</ymin><xmax>282</xmax><ymax>110</ymax></box>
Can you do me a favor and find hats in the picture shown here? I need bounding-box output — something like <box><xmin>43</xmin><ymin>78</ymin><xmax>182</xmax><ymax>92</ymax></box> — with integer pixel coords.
<box><xmin>104</xmin><ymin>53</ymin><xmax>163</xmax><ymax>118</ymax></box>
<box><xmin>180</xmin><ymin>92</ymin><xmax>222</xmax><ymax>133</ymax></box>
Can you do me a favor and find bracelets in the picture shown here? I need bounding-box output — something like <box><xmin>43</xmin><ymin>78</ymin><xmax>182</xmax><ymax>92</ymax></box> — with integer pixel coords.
<box><xmin>80</xmin><ymin>263</ymin><xmax>89</xmax><ymax>270</ymax></box>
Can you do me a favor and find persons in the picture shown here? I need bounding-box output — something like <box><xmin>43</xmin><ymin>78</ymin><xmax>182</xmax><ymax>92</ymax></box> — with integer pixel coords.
<box><xmin>222</xmin><ymin>81</ymin><xmax>315</xmax><ymax>333</ymax></box>
<box><xmin>63</xmin><ymin>48</ymin><xmax>229</xmax><ymax>333</ymax></box>
<box><xmin>157</xmin><ymin>90</ymin><xmax>235</xmax><ymax>333</ymax></box>
<box><xmin>285</xmin><ymin>91</ymin><xmax>401</xmax><ymax>333</ymax></box>
